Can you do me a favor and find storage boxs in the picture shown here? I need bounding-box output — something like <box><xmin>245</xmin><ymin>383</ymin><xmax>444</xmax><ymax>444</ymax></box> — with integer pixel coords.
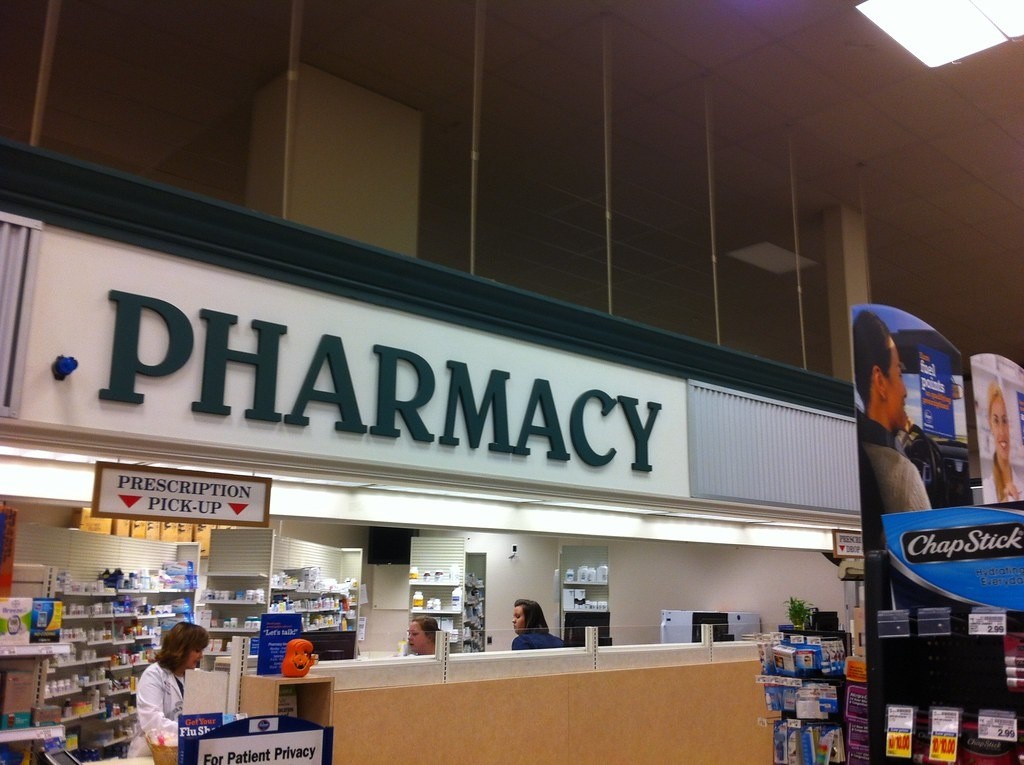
<box><xmin>0</xmin><ymin>598</ymin><xmax>62</xmax><ymax>730</ymax></box>
<box><xmin>70</xmin><ymin>506</ymin><xmax>235</xmax><ymax>557</ymax></box>
<box><xmin>204</xmin><ymin>637</ymin><xmax>259</xmax><ymax>676</ymax></box>
<box><xmin>752</xmin><ymin>634</ymin><xmax>845</xmax><ymax>676</ymax></box>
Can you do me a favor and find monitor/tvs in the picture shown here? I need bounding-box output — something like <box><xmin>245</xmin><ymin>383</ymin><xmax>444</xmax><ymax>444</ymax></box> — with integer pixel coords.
<box><xmin>43</xmin><ymin>749</ymin><xmax>82</xmax><ymax>765</ymax></box>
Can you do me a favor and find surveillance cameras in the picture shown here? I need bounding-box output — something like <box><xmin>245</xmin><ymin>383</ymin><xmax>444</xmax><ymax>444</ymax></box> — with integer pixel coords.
<box><xmin>512</xmin><ymin>544</ymin><xmax>518</xmax><ymax>554</ymax></box>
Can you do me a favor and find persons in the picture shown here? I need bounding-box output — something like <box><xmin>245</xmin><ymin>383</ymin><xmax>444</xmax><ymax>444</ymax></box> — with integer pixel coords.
<box><xmin>127</xmin><ymin>622</ymin><xmax>209</xmax><ymax>758</ymax></box>
<box><xmin>852</xmin><ymin>311</ymin><xmax>932</xmax><ymax>512</ymax></box>
<box><xmin>982</xmin><ymin>384</ymin><xmax>1024</xmax><ymax>504</ymax></box>
<box><xmin>408</xmin><ymin>618</ymin><xmax>441</xmax><ymax>654</ymax></box>
<box><xmin>512</xmin><ymin>599</ymin><xmax>564</xmax><ymax>650</ymax></box>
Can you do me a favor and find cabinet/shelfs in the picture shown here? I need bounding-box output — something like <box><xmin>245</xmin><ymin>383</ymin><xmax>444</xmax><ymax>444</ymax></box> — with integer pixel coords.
<box><xmin>12</xmin><ymin>522</ymin><xmax>610</xmax><ymax>750</ymax></box>
<box><xmin>0</xmin><ymin>646</ymin><xmax>70</xmax><ymax>743</ymax></box>
